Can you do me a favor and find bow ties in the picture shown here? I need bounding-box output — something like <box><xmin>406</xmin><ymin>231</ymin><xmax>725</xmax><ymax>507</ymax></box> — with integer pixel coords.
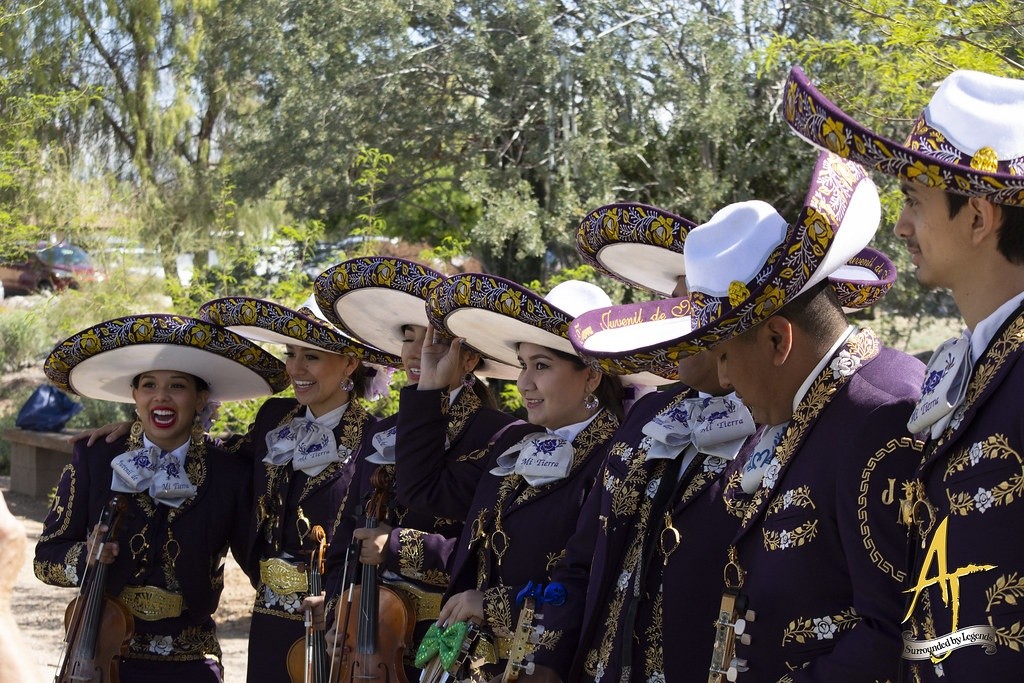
<box><xmin>741</xmin><ymin>421</ymin><xmax>790</xmax><ymax>493</ymax></box>
<box><xmin>489</xmin><ymin>432</ymin><xmax>573</xmax><ymax>487</ymax></box>
<box><xmin>907</xmin><ymin>337</ymin><xmax>972</xmax><ymax>439</ymax></box>
<box><xmin>110</xmin><ymin>446</ymin><xmax>194</xmax><ymax>508</ymax></box>
<box><xmin>365</xmin><ymin>426</ymin><xmax>396</xmax><ymax>464</ymax></box>
<box><xmin>262</xmin><ymin>418</ymin><xmax>339</xmax><ymax>477</ymax></box>
<box><xmin>641</xmin><ymin>398</ymin><xmax>756</xmax><ymax>460</ymax></box>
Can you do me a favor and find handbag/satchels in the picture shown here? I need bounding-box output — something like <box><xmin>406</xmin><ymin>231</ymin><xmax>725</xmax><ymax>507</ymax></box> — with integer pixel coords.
<box><xmin>15</xmin><ymin>383</ymin><xmax>83</xmax><ymax>433</ymax></box>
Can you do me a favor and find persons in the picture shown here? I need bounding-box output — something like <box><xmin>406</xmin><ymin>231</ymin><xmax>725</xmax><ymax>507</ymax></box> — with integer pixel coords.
<box><xmin>68</xmin><ymin>293</ymin><xmax>403</xmax><ymax>683</ymax></box>
<box><xmin>395</xmin><ymin>273</ymin><xmax>681</xmax><ymax>683</ymax></box>
<box><xmin>567</xmin><ymin>202</ymin><xmax>897</xmax><ymax>683</ymax></box>
<box><xmin>313</xmin><ymin>256</ymin><xmax>522</xmax><ymax>683</ymax></box>
<box><xmin>33</xmin><ymin>314</ymin><xmax>292</xmax><ymax>683</ymax></box>
<box><xmin>568</xmin><ymin>151</ymin><xmax>926</xmax><ymax>683</ymax></box>
<box><xmin>0</xmin><ymin>491</ymin><xmax>45</xmax><ymax>683</ymax></box>
<box><xmin>782</xmin><ymin>68</ymin><xmax>1024</xmax><ymax>683</ymax></box>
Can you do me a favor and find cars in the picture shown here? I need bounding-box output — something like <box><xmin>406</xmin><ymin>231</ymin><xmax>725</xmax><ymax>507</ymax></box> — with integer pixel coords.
<box><xmin>0</xmin><ymin>240</ymin><xmax>110</xmax><ymax>298</ymax></box>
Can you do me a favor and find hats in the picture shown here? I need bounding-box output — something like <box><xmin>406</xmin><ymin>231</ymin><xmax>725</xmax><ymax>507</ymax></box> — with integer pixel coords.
<box><xmin>575</xmin><ymin>204</ymin><xmax>897</xmax><ymax>314</ymax></box>
<box><xmin>314</xmin><ymin>257</ymin><xmax>523</xmax><ymax>380</ymax></box>
<box><xmin>199</xmin><ymin>293</ymin><xmax>405</xmax><ymax>371</ymax></box>
<box><xmin>783</xmin><ymin>66</ymin><xmax>1024</xmax><ymax>207</ymax></box>
<box><xmin>425</xmin><ymin>273</ymin><xmax>680</xmax><ymax>386</ymax></box>
<box><xmin>43</xmin><ymin>314</ymin><xmax>292</xmax><ymax>404</ymax></box>
<box><xmin>569</xmin><ymin>151</ymin><xmax>881</xmax><ymax>376</ymax></box>
